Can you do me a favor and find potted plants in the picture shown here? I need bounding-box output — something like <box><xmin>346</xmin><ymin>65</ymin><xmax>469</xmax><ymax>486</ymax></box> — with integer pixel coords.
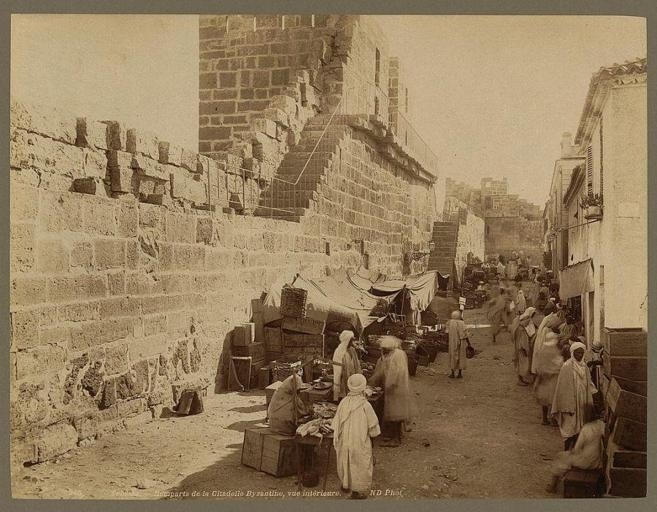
<box><xmin>581</xmin><ymin>192</ymin><xmax>605</xmax><ymax>219</ymax></box>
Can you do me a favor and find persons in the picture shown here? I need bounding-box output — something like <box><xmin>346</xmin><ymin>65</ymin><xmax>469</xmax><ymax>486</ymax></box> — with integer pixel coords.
<box><xmin>267</xmin><ymin>373</ymin><xmax>306</xmax><ymax>434</ymax></box>
<box><xmin>332</xmin><ymin>329</ymin><xmax>363</xmax><ymax>403</ymax></box>
<box><xmin>367</xmin><ymin>335</ymin><xmax>410</xmax><ymax>448</ymax></box>
<box><xmin>486</xmin><ymin>254</ymin><xmax>611</xmax><ymax>495</ymax></box>
<box><xmin>445</xmin><ymin>310</ymin><xmax>471</xmax><ymax>379</ymax></box>
<box><xmin>330</xmin><ymin>373</ymin><xmax>383</xmax><ymax>499</ymax></box>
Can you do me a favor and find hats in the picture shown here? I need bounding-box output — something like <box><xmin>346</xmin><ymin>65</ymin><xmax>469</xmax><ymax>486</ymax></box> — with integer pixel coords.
<box><xmin>346</xmin><ymin>374</ymin><xmax>367</xmax><ymax>393</ymax></box>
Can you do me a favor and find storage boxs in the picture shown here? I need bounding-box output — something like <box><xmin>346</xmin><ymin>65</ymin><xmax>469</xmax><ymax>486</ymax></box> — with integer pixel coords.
<box><xmin>226</xmin><ymin>285</ymin><xmax>429</xmax><ymax>474</ymax></box>
<box><xmin>588</xmin><ymin>326</ymin><xmax>648</xmax><ymax>497</ymax></box>
